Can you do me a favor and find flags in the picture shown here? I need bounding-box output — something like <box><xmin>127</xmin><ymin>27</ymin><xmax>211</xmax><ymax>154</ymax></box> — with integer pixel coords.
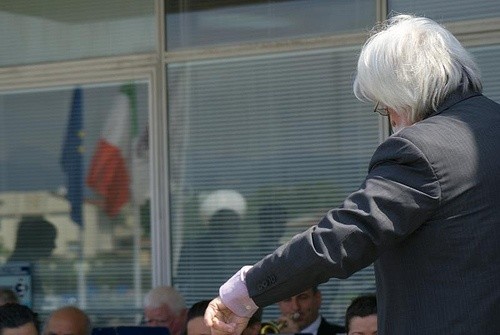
<box><xmin>60</xmin><ymin>88</ymin><xmax>84</xmax><ymax>229</ymax></box>
<box><xmin>88</xmin><ymin>84</ymin><xmax>140</xmax><ymax>216</ymax></box>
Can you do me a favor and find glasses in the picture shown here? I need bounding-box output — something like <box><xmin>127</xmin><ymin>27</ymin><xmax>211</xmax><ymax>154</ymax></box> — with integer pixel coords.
<box><xmin>373</xmin><ymin>101</ymin><xmax>390</xmax><ymax>116</ymax></box>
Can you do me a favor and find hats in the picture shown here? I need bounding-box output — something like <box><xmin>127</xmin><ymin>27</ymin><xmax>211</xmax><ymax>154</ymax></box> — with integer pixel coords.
<box><xmin>201</xmin><ymin>189</ymin><xmax>247</xmax><ymax>226</ymax></box>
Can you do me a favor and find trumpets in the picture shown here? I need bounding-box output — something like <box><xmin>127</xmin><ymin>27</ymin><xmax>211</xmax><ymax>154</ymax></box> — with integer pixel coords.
<box><xmin>260</xmin><ymin>312</ymin><xmax>301</xmax><ymax>335</ymax></box>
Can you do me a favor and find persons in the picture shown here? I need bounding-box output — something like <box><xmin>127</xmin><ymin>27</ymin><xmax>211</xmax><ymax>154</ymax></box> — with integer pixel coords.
<box><xmin>0</xmin><ymin>188</ymin><xmax>289</xmax><ymax>320</ymax></box>
<box><xmin>205</xmin><ymin>15</ymin><xmax>500</xmax><ymax>335</ymax></box>
<box><xmin>0</xmin><ymin>284</ymin><xmax>379</xmax><ymax>335</ymax></box>
